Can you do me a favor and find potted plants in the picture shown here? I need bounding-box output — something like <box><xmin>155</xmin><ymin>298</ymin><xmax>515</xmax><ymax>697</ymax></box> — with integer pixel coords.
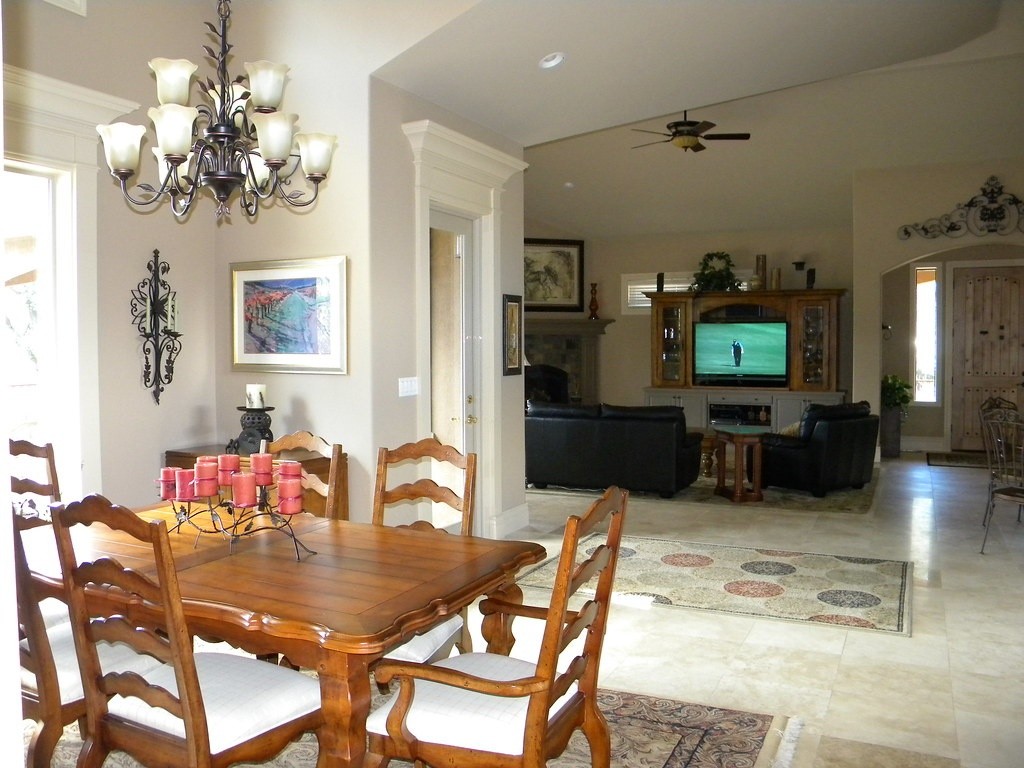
<box><xmin>880</xmin><ymin>374</ymin><xmax>913</xmax><ymax>457</ymax></box>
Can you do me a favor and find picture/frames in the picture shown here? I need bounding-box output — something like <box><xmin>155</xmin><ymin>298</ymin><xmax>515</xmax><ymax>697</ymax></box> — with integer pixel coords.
<box><xmin>230</xmin><ymin>254</ymin><xmax>347</xmax><ymax>377</ymax></box>
<box><xmin>524</xmin><ymin>237</ymin><xmax>585</xmax><ymax>312</ymax></box>
<box><xmin>502</xmin><ymin>294</ymin><xmax>522</xmax><ymax>375</ymax></box>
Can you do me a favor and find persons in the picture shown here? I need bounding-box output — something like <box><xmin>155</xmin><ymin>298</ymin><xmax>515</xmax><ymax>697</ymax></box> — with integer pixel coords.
<box><xmin>731</xmin><ymin>339</ymin><xmax>744</xmax><ymax>367</ymax></box>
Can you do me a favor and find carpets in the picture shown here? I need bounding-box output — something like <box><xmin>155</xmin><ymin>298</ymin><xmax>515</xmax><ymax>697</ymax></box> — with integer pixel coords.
<box><xmin>927</xmin><ymin>452</ymin><xmax>1021</xmax><ymax>469</ymax></box>
<box><xmin>515</xmin><ymin>531</ymin><xmax>915</xmax><ymax>636</ymax></box>
<box><xmin>22</xmin><ymin>668</ymin><xmax>805</xmax><ymax>768</ymax></box>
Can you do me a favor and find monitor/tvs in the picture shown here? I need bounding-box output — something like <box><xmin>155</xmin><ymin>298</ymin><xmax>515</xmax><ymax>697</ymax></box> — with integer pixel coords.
<box><xmin>692</xmin><ymin>320</ymin><xmax>791</xmax><ymax>388</ymax></box>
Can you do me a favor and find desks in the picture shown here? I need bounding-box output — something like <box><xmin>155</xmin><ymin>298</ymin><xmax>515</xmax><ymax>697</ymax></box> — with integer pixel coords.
<box><xmin>714</xmin><ymin>425</ymin><xmax>764</xmax><ymax>503</ymax></box>
<box><xmin>26</xmin><ymin>499</ymin><xmax>547</xmax><ymax>768</ymax></box>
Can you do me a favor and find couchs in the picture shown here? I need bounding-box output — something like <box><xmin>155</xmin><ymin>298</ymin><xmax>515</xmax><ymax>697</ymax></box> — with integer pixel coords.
<box><xmin>525</xmin><ymin>363</ymin><xmax>703</xmax><ymax>499</ymax></box>
<box><xmin>746</xmin><ymin>399</ymin><xmax>880</xmax><ymax>498</ymax></box>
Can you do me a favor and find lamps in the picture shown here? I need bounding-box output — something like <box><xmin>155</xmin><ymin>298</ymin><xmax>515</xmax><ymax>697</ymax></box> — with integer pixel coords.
<box><xmin>96</xmin><ymin>0</ymin><xmax>339</xmax><ymax>218</ymax></box>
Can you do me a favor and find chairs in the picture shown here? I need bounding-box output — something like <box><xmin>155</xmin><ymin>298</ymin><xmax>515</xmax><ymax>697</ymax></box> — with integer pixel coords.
<box><xmin>256</xmin><ymin>431</ymin><xmax>343</xmax><ymax>664</ymax></box>
<box><xmin>48</xmin><ymin>493</ymin><xmax>322</xmax><ymax>768</ymax></box>
<box><xmin>278</xmin><ymin>439</ymin><xmax>477</xmax><ymax>671</ymax></box>
<box><xmin>12</xmin><ymin>504</ymin><xmax>173</xmax><ymax>768</ymax></box>
<box><xmin>978</xmin><ymin>397</ymin><xmax>1024</xmax><ymax>554</ymax></box>
<box><xmin>363</xmin><ymin>486</ymin><xmax>629</xmax><ymax>768</ymax></box>
<box><xmin>9</xmin><ymin>440</ymin><xmax>72</xmax><ymax>638</ymax></box>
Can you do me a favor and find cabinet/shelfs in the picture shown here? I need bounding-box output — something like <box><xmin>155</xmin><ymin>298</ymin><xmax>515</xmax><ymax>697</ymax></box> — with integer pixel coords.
<box><xmin>641</xmin><ymin>386</ymin><xmax>847</xmax><ymax>436</ymax></box>
<box><xmin>165</xmin><ymin>445</ymin><xmax>349</xmax><ymax>520</ymax></box>
<box><xmin>641</xmin><ymin>289</ymin><xmax>847</xmax><ymax>392</ymax></box>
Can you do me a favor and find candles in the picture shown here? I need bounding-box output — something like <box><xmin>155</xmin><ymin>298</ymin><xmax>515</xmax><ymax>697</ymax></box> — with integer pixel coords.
<box><xmin>174</xmin><ymin>298</ymin><xmax>177</xmax><ymax>331</ymax></box>
<box><xmin>159</xmin><ymin>455</ymin><xmax>303</xmax><ymax>513</ymax></box>
<box><xmin>770</xmin><ymin>266</ymin><xmax>781</xmax><ymax>289</ymax></box>
<box><xmin>168</xmin><ymin>294</ymin><xmax>171</xmax><ymax>329</ymax></box>
<box><xmin>146</xmin><ymin>296</ymin><xmax>151</xmax><ymax>332</ymax></box>
<box><xmin>246</xmin><ymin>382</ymin><xmax>266</xmax><ymax>408</ymax></box>
